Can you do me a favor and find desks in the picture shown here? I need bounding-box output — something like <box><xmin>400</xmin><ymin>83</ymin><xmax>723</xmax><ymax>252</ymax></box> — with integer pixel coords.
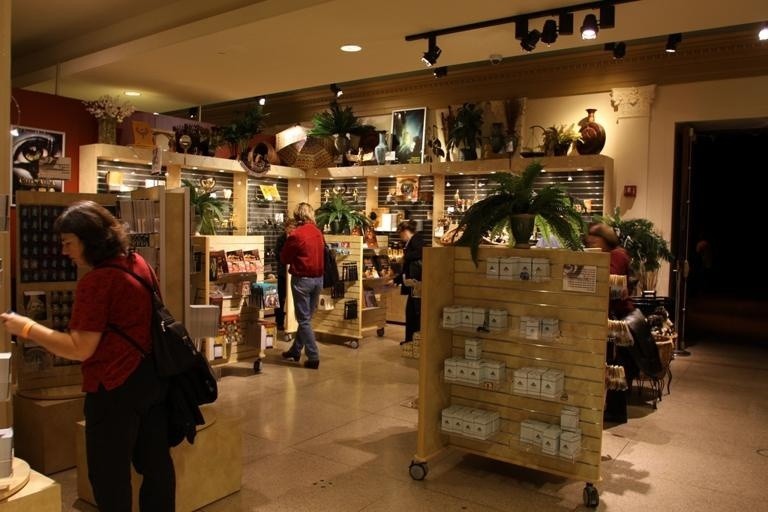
<box><xmin>1</xmin><ymin>456</ymin><xmax>63</xmax><ymax>511</ymax></box>
<box><xmin>77</xmin><ymin>406</ymin><xmax>243</xmax><ymax>511</ymax></box>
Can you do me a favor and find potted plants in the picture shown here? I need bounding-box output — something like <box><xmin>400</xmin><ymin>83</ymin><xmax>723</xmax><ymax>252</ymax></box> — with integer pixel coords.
<box><xmin>529</xmin><ymin>120</ymin><xmax>582</xmax><ymax>156</ymax></box>
<box><xmin>309</xmin><ymin>102</ymin><xmax>366</xmax><ymax>153</ymax></box>
<box><xmin>444</xmin><ymin>102</ymin><xmax>483</xmax><ymax>161</ymax></box>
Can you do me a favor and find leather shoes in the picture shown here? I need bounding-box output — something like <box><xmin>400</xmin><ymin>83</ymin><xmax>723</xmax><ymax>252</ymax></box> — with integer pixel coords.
<box><xmin>282</xmin><ymin>351</ymin><xmax>301</xmax><ymax>362</ymax></box>
<box><xmin>304</xmin><ymin>359</ymin><xmax>320</xmax><ymax>369</ymax></box>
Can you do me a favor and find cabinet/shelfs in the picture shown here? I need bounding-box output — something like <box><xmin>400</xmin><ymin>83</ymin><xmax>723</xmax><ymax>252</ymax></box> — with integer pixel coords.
<box><xmin>79</xmin><ymin>148</ymin><xmax>189</xmax><ymax>195</ymax></box>
<box><xmin>192</xmin><ymin>154</ymin><xmax>418</xmax><ymax>319</ymax></box>
<box><xmin>15</xmin><ymin>190</ymin><xmax>186</xmax><ymax>475</ymax></box>
<box><xmin>405</xmin><ymin>156</ymin><xmax>618</xmax><ymax>254</ymax></box>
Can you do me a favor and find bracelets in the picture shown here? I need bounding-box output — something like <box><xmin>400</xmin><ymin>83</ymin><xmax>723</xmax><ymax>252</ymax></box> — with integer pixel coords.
<box><xmin>22</xmin><ymin>320</ymin><xmax>35</xmax><ymax>339</ymax></box>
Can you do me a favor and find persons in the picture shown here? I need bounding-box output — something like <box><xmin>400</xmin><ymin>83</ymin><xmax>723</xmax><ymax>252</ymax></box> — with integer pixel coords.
<box><xmin>387</xmin><ymin>220</ymin><xmax>424</xmax><ymax>346</ymax></box>
<box><xmin>275</xmin><ymin>219</ymin><xmax>299</xmax><ymax>341</ymax></box>
<box><xmin>0</xmin><ymin>200</ymin><xmax>175</xmax><ymax>511</ymax></box>
<box><xmin>588</xmin><ymin>223</ymin><xmax>634</xmax><ymax>424</ymax></box>
<box><xmin>279</xmin><ymin>203</ymin><xmax>325</xmax><ymax>369</ymax></box>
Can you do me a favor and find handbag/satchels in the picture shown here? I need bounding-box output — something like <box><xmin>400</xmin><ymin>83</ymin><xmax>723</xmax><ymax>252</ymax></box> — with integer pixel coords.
<box><xmin>91</xmin><ymin>262</ymin><xmax>218</xmax><ymax>406</ymax></box>
<box><xmin>322</xmin><ymin>243</ymin><xmax>339</xmax><ymax>288</ymax></box>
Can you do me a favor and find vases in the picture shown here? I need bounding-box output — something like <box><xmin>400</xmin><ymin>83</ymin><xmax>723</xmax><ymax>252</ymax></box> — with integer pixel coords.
<box><xmin>575</xmin><ymin>106</ymin><xmax>606</xmax><ymax>154</ymax></box>
<box><xmin>374</xmin><ymin>133</ymin><xmax>388</xmax><ymax>165</ymax></box>
<box><xmin>98</xmin><ymin>119</ymin><xmax>119</xmax><ymax>144</ymax></box>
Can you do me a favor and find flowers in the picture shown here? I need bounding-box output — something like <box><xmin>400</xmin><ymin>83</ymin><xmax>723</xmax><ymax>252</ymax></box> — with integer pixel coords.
<box><xmin>82</xmin><ymin>93</ymin><xmax>138</xmax><ymax>122</ymax></box>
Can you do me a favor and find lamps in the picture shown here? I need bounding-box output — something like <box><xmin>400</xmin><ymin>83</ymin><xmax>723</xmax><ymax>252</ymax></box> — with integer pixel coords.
<box><xmin>402</xmin><ymin>0</ymin><xmax>618</xmax><ymax>69</ymax></box>
<box><xmin>185</xmin><ymin>34</ymin><xmax>689</xmax><ymax>123</ymax></box>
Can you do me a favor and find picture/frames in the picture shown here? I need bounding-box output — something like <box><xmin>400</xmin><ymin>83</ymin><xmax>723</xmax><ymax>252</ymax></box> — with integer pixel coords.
<box><xmin>389</xmin><ymin>106</ymin><xmax>427</xmax><ymax>165</ymax></box>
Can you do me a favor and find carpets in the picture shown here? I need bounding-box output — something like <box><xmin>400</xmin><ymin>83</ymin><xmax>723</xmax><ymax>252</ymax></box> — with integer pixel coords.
<box><xmin>671</xmin><ymin>332</ymin><xmax>768</xmax><ymax>370</ymax></box>
